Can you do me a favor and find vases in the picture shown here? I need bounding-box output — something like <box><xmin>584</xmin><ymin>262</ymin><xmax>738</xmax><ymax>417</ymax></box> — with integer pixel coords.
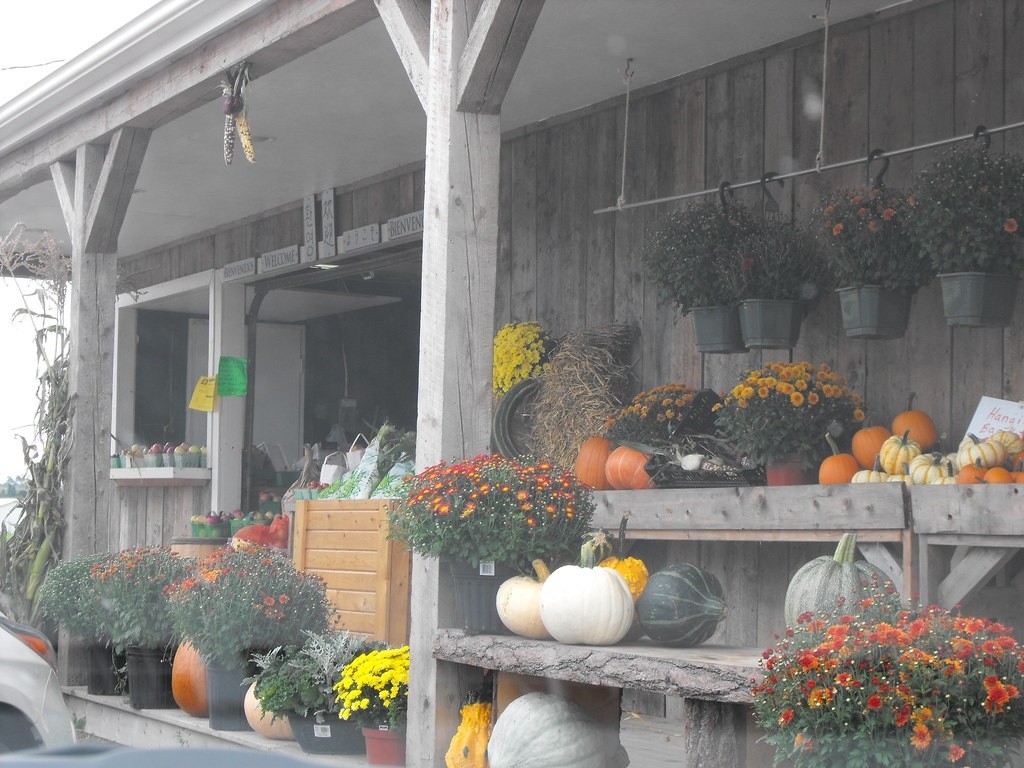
<box><xmin>835</xmin><ymin>285</ymin><xmax>912</xmax><ymax>340</ymax></box>
<box><xmin>937</xmin><ymin>274</ymin><xmax>1016</xmax><ymax>327</ymax></box>
<box><xmin>85</xmin><ymin>633</ymin><xmax>126</xmax><ymax>695</ymax></box>
<box><xmin>126</xmin><ymin>646</ymin><xmax>177</xmax><ymax>710</ymax></box>
<box><xmin>687</xmin><ymin>306</ymin><xmax>750</xmax><ymax>354</ymax></box>
<box><xmin>769</xmin><ymin>456</ymin><xmax>812</xmax><ymax>484</ymax></box>
<box><xmin>734</xmin><ymin>299</ymin><xmax>801</xmax><ymax>349</ymax></box>
<box><xmin>204</xmin><ymin>662</ymin><xmax>251</xmax><ymax>732</ymax></box>
<box><xmin>360</xmin><ymin>727</ymin><xmax>406</xmax><ymax>768</ymax></box>
<box><xmin>448</xmin><ymin>559</ymin><xmax>519</xmax><ymax>635</ymax></box>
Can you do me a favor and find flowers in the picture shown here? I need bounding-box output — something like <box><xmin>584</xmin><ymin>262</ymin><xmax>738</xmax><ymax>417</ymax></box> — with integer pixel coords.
<box><xmin>630</xmin><ymin>145</ymin><xmax>1024</xmax><ymax>324</ymax></box>
<box><xmin>333</xmin><ymin>647</ymin><xmax>410</xmax><ymax>730</ymax></box>
<box><xmin>491</xmin><ymin>319</ymin><xmax>556</xmax><ymax>399</ymax></box>
<box><xmin>385</xmin><ymin>451</ymin><xmax>599</xmax><ymax>573</ymax></box>
<box><xmin>749</xmin><ymin>589</ymin><xmax>1024</xmax><ymax>768</ymax></box>
<box><xmin>37</xmin><ymin>537</ymin><xmax>347</xmax><ymax>664</ymax></box>
<box><xmin>607</xmin><ymin>359</ymin><xmax>866</xmax><ymax>469</ymax></box>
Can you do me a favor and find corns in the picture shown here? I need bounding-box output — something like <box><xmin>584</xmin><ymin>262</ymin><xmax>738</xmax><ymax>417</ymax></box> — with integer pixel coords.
<box><xmin>699</xmin><ymin>460</ymin><xmax>740</xmax><ymax>476</ymax></box>
<box><xmin>32</xmin><ymin>531</ymin><xmax>55</xmax><ymax>577</ymax></box>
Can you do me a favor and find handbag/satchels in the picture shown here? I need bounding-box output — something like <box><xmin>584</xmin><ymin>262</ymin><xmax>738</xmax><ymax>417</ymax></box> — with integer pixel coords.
<box><xmin>345</xmin><ymin>433</ymin><xmax>371</xmax><ymax>471</ymax></box>
<box><xmin>318</xmin><ymin>450</ymin><xmax>348</xmax><ymax>488</ymax></box>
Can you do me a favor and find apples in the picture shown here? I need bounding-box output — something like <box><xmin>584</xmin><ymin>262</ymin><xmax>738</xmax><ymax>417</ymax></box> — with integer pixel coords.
<box><xmin>305</xmin><ymin>480</ymin><xmax>329</xmax><ymax>490</ymax></box>
<box><xmin>191</xmin><ymin>510</ymin><xmax>283</xmax><ymax>539</ymax></box>
<box><xmin>259</xmin><ymin>492</ymin><xmax>283</xmax><ymax>503</ymax></box>
<box><xmin>122</xmin><ymin>442</ymin><xmax>208</xmax><ymax>455</ymax></box>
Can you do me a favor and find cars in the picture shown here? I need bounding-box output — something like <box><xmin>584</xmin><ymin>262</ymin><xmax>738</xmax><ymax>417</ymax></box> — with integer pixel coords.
<box><xmin>0</xmin><ymin>611</ymin><xmax>319</xmax><ymax>768</ymax></box>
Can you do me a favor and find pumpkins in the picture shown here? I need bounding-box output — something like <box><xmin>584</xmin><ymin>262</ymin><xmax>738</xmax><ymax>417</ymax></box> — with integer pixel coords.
<box><xmin>495</xmin><ymin>513</ymin><xmax>728</xmax><ymax>646</ymax></box>
<box><xmin>574</xmin><ymin>435</ymin><xmax>614</xmax><ymax>490</ymax></box>
<box><xmin>487</xmin><ymin>693</ymin><xmax>607</xmax><ymax>768</ymax></box>
<box><xmin>171</xmin><ymin>637</ymin><xmax>209</xmax><ymax>717</ymax></box>
<box><xmin>243</xmin><ymin>682</ymin><xmax>297</xmax><ymax>740</ymax></box>
<box><xmin>819</xmin><ymin>391</ymin><xmax>1024</xmax><ymax>483</ymax></box>
<box><xmin>605</xmin><ymin>445</ymin><xmax>657</xmax><ymax>490</ymax></box>
<box><xmin>785</xmin><ymin>532</ymin><xmax>900</xmax><ymax>634</ymax></box>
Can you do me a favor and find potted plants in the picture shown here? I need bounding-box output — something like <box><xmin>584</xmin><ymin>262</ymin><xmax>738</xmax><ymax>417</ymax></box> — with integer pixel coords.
<box><xmin>241</xmin><ymin>625</ymin><xmax>397</xmax><ymax>757</ymax></box>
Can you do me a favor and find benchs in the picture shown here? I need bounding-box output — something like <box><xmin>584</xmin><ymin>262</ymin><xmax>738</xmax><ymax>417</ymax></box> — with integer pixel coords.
<box><xmin>434</xmin><ymin>629</ymin><xmax>775</xmax><ymax>768</ymax></box>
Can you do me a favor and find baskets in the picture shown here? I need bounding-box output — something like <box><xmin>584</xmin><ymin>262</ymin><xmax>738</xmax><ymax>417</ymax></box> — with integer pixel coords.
<box><xmin>189</xmin><ymin>510</ymin><xmax>230</xmax><ymax>538</ymax></box>
<box><xmin>110</xmin><ymin>453</ymin><xmax>208</xmax><ymax>468</ymax></box>
<box><xmin>644</xmin><ymin>388</ymin><xmax>767</xmax><ymax>487</ymax></box>
<box><xmin>231</xmin><ymin>513</ymin><xmax>266</xmax><ymax>539</ymax></box>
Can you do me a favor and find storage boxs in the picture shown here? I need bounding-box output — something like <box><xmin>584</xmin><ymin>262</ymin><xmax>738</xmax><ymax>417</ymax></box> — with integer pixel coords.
<box><xmin>190</xmin><ymin>471</ymin><xmax>299</xmax><ymax>537</ymax></box>
<box><xmin>291</xmin><ymin>499</ymin><xmax>412</xmax><ymax>649</ymax></box>
<box><xmin>110</xmin><ymin>452</ymin><xmax>207</xmax><ymax>468</ymax></box>
<box><xmin>644</xmin><ymin>388</ymin><xmax>767</xmax><ymax>488</ymax></box>
